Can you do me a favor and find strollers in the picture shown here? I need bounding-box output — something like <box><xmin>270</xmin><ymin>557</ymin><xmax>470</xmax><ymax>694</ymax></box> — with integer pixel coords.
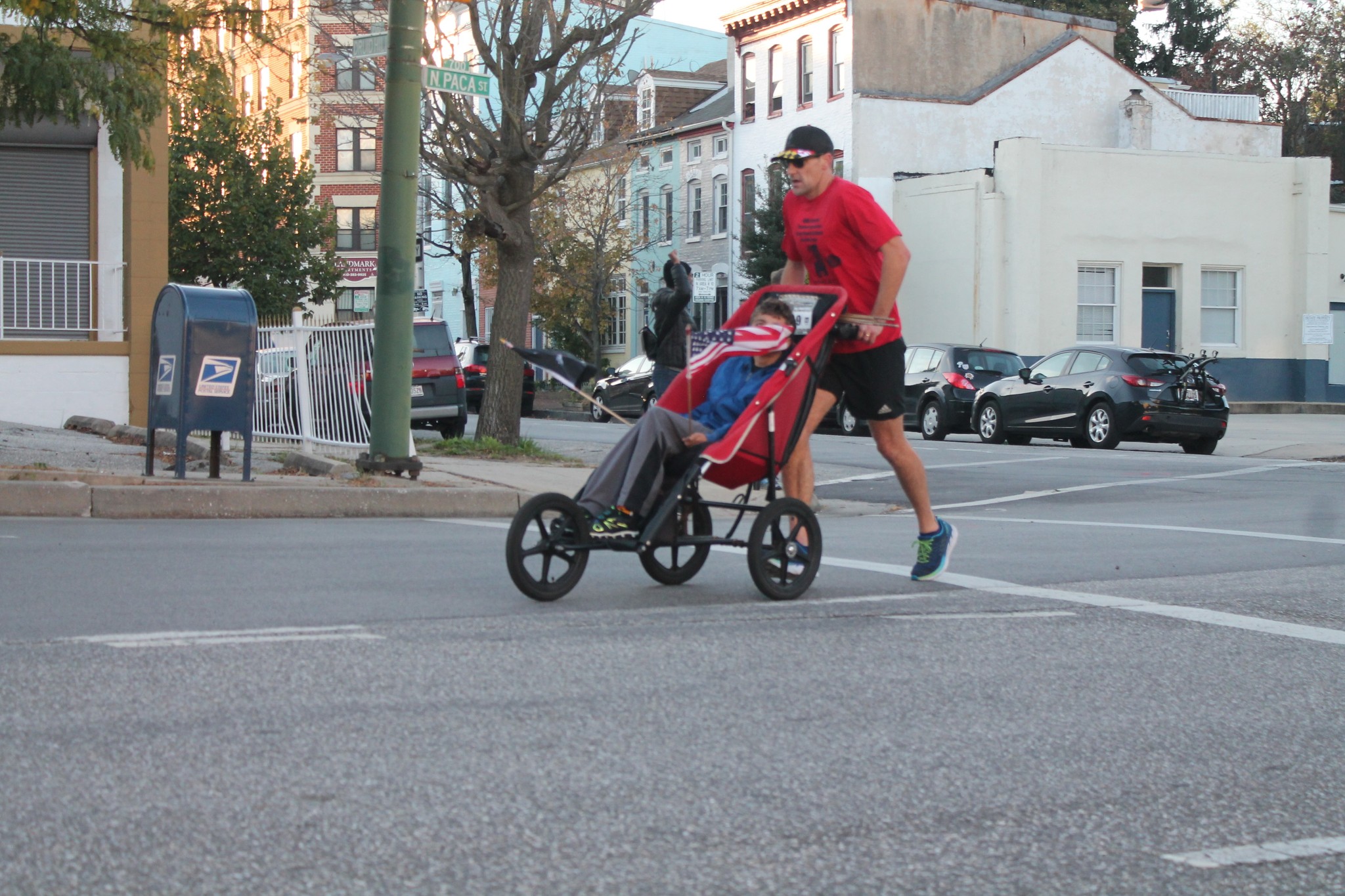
<box><xmin>506</xmin><ymin>285</ymin><xmax>860</xmax><ymax>603</ymax></box>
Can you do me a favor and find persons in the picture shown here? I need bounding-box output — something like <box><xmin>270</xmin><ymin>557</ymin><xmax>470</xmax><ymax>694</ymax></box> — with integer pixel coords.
<box><xmin>650</xmin><ymin>248</ymin><xmax>694</xmax><ymax>399</ymax></box>
<box><xmin>766</xmin><ymin>123</ymin><xmax>957</xmax><ymax>582</ymax></box>
<box><xmin>555</xmin><ymin>297</ymin><xmax>797</xmax><ymax>540</ymax></box>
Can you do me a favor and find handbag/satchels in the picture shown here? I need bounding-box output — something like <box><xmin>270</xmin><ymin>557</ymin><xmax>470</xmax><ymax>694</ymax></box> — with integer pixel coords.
<box><xmin>641</xmin><ymin>326</ymin><xmax>659</xmax><ymax>362</ymax></box>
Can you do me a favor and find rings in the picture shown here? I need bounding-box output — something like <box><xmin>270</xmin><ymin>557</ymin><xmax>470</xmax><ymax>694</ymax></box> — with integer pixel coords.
<box><xmin>865</xmin><ymin>334</ymin><xmax>870</xmax><ymax>337</ymax></box>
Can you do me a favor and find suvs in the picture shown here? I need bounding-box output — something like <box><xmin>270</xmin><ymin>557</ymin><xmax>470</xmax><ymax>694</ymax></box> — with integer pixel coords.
<box><xmin>254</xmin><ymin>319</ymin><xmax>469</xmax><ymax>443</ymax></box>
<box><xmin>452</xmin><ymin>336</ymin><xmax>537</xmax><ymax>416</ymax></box>
<box><xmin>527</xmin><ymin>347</ymin><xmax>595</xmax><ymax>387</ymax></box>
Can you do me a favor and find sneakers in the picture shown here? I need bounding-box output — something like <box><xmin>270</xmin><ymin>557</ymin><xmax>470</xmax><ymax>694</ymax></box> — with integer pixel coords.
<box><xmin>910</xmin><ymin>516</ymin><xmax>958</xmax><ymax>584</ymax></box>
<box><xmin>764</xmin><ymin>539</ymin><xmax>819</xmax><ymax>580</ymax></box>
<box><xmin>584</xmin><ymin>507</ymin><xmax>640</xmax><ymax>539</ymax></box>
<box><xmin>550</xmin><ymin>517</ymin><xmax>591</xmax><ymax>533</ymax></box>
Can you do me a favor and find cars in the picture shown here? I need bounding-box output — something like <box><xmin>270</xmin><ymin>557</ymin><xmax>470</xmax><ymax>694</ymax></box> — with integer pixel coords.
<box><xmin>591</xmin><ymin>355</ymin><xmax>662</xmax><ymax>425</ymax></box>
<box><xmin>816</xmin><ymin>339</ymin><xmax>1030</xmax><ymax>444</ymax></box>
<box><xmin>970</xmin><ymin>342</ymin><xmax>1229</xmax><ymax>453</ymax></box>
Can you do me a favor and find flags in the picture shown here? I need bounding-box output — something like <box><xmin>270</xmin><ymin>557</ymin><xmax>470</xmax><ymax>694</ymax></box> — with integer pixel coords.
<box><xmin>685</xmin><ymin>324</ymin><xmax>795</xmax><ymax>380</ymax></box>
<box><xmin>511</xmin><ymin>344</ymin><xmax>599</xmax><ymax>391</ymax></box>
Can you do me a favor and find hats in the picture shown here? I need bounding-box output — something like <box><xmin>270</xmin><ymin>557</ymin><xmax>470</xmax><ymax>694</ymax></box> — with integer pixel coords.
<box><xmin>770</xmin><ymin>125</ymin><xmax>833</xmax><ymax>163</ymax></box>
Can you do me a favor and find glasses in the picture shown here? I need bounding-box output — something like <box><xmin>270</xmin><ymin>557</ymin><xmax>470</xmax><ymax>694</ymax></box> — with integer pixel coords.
<box><xmin>781</xmin><ymin>152</ymin><xmax>824</xmax><ymax>169</ymax></box>
